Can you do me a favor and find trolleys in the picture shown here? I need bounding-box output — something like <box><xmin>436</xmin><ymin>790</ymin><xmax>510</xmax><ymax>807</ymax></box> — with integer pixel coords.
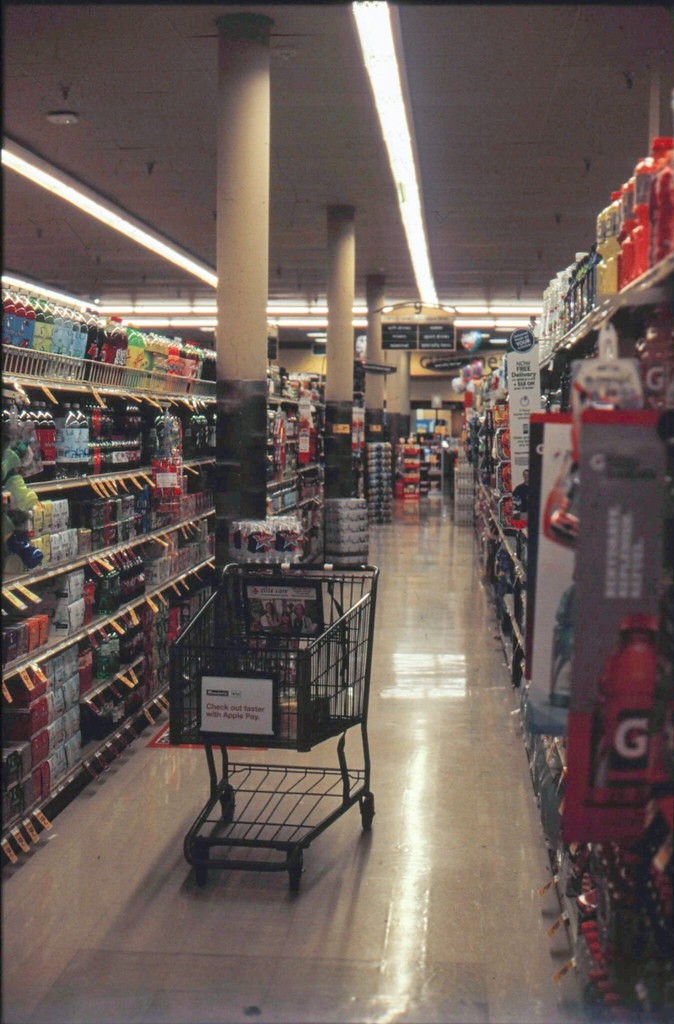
<box><xmin>170</xmin><ymin>559</ymin><xmax>382</xmax><ymax>892</ymax></box>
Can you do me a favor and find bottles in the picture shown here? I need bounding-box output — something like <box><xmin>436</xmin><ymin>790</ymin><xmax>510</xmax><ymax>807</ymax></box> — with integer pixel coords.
<box><xmin>79</xmin><ymin>554</ymin><xmax>145</xmax><ymax>697</ymax></box>
<box><xmin>229</xmin><ymin>514</ymin><xmax>304</xmax><ymax>565</ymax></box>
<box><xmin>1</xmin><ymin>284</ymin><xmax>219</xmax><ymax>480</ymax></box>
<box><xmin>539</xmin><ymin>132</ymin><xmax>674</xmax><ymax>431</ymax></box>
<box><xmin>584</xmin><ymin>614</ymin><xmax>658</xmax><ymax>811</ymax></box>
<box><xmin>552</xmin><ymin>839</ymin><xmax>674</xmax><ymax>1024</ymax></box>
